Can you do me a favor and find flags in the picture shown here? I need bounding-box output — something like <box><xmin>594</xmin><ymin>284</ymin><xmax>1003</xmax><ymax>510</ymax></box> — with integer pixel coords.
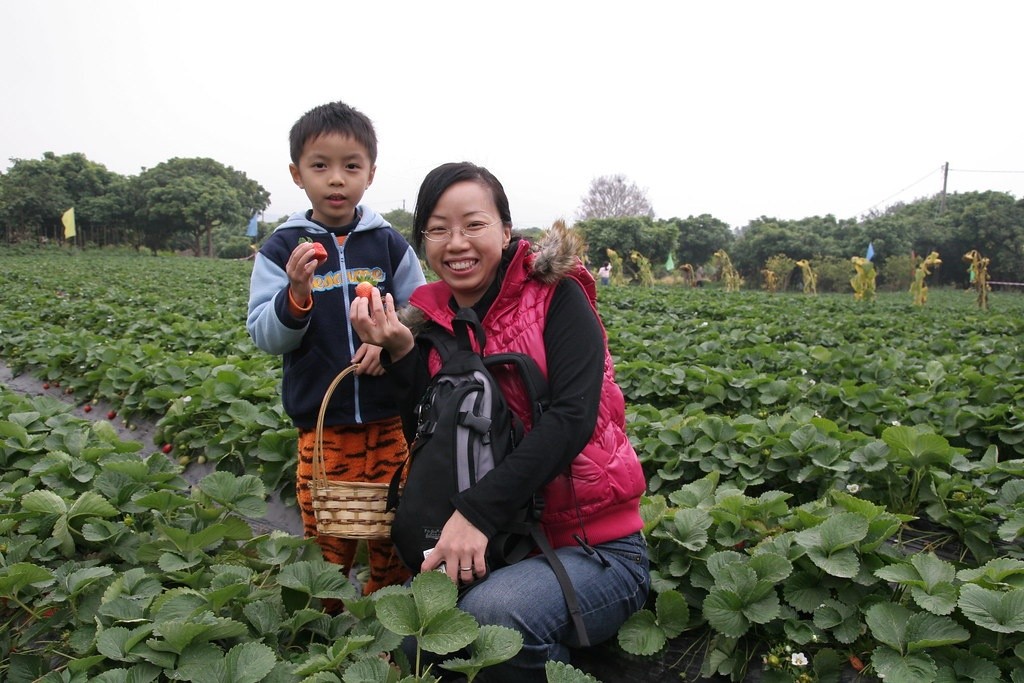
<box><xmin>911</xmin><ymin>250</ymin><xmax>916</xmax><ymax>276</ymax></box>
<box><xmin>969</xmin><ymin>264</ymin><xmax>976</xmax><ymax>284</ymax></box>
<box><xmin>62</xmin><ymin>207</ymin><xmax>76</xmax><ymax>239</ymax></box>
<box><xmin>246</xmin><ymin>210</ymin><xmax>257</xmax><ymax>237</ymax></box>
<box><xmin>865</xmin><ymin>243</ymin><xmax>875</xmax><ymax>263</ymax></box>
<box><xmin>664</xmin><ymin>253</ymin><xmax>675</xmax><ymax>271</ymax></box>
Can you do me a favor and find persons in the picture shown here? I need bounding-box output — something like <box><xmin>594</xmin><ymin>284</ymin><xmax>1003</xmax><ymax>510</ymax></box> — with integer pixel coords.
<box><xmin>246</xmin><ymin>103</ymin><xmax>428</xmax><ymax>622</ymax></box>
<box><xmin>597</xmin><ymin>261</ymin><xmax>612</xmax><ymax>287</ymax></box>
<box><xmin>350</xmin><ymin>163</ymin><xmax>650</xmax><ymax>683</ymax></box>
<box><xmin>696</xmin><ymin>263</ymin><xmax>706</xmax><ymax>288</ymax></box>
<box><xmin>581</xmin><ymin>243</ymin><xmax>591</xmax><ymax>270</ymax></box>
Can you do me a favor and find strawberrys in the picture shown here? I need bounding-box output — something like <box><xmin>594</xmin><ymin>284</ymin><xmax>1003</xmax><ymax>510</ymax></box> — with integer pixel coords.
<box><xmin>42</xmin><ymin>382</ymin><xmax>174</xmax><ymax>454</ymax></box>
<box><xmin>305</xmin><ymin>242</ymin><xmax>327</xmax><ymax>262</ymax></box>
<box><xmin>356</xmin><ymin>281</ymin><xmax>374</xmax><ymax>299</ymax></box>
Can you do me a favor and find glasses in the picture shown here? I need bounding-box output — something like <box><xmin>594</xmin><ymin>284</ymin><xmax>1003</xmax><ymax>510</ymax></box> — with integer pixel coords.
<box><xmin>421</xmin><ymin>218</ymin><xmax>502</xmax><ymax>243</ymax></box>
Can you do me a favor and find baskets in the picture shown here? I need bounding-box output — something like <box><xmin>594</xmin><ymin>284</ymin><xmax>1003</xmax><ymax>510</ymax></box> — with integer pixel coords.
<box><xmin>307</xmin><ymin>364</ymin><xmax>409</xmax><ymax>540</ymax></box>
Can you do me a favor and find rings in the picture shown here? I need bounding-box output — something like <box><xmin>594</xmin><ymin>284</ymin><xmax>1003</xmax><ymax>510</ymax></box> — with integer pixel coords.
<box><xmin>459</xmin><ymin>567</ymin><xmax>472</xmax><ymax>571</ymax></box>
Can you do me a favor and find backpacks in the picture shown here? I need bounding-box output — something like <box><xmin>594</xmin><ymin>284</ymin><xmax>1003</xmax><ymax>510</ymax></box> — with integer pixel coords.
<box><xmin>385</xmin><ymin>306</ymin><xmax>546</xmax><ymax>588</ymax></box>
